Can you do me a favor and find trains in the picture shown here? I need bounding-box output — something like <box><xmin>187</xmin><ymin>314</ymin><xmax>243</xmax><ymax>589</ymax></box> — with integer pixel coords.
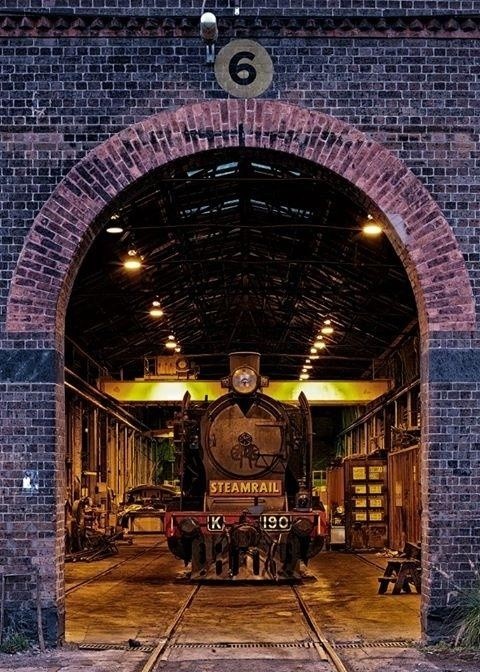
<box><xmin>156</xmin><ymin>351</ymin><xmax>332</xmax><ymax>574</ymax></box>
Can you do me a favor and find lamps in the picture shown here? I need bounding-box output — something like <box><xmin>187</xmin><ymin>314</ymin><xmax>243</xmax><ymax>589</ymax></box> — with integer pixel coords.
<box><xmin>103</xmin><ymin>214</ymin><xmax>129</xmax><ymax>235</ymax></box>
<box><xmin>122</xmin><ymin>249</ymin><xmax>144</xmax><ymax>270</ymax></box>
<box><xmin>356</xmin><ymin>212</ymin><xmax>388</xmax><ymax>238</ymax></box>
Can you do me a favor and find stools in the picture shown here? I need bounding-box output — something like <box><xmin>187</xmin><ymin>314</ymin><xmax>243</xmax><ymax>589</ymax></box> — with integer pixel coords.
<box><xmin>373</xmin><ymin>558</ymin><xmax>421</xmax><ymax>598</ymax></box>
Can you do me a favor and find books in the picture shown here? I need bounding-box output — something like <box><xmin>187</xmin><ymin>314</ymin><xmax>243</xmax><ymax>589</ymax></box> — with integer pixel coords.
<box><xmin>369</xmin><ymin>472</ymin><xmax>379</xmax><ymax>480</ymax></box>
<box><xmin>355</xmin><ymin>483</ymin><xmax>366</xmax><ymax>493</ymax></box>
<box><xmin>356</xmin><ymin>513</ymin><xmax>366</xmax><ymax>520</ymax></box>
<box><xmin>353</xmin><ymin>468</ymin><xmax>366</xmax><ymax>480</ymax></box>
<box><xmin>369</xmin><ymin>484</ymin><xmax>381</xmax><ymax>493</ymax></box>
<box><xmin>369</xmin><ymin>500</ymin><xmax>381</xmax><ymax>507</ymax></box>
<box><xmin>354</xmin><ymin>497</ymin><xmax>367</xmax><ymax>507</ymax></box>
<box><xmin>369</xmin><ymin>513</ymin><xmax>381</xmax><ymax>520</ymax></box>
<box><xmin>369</xmin><ymin>467</ymin><xmax>382</xmax><ymax>472</ymax></box>
